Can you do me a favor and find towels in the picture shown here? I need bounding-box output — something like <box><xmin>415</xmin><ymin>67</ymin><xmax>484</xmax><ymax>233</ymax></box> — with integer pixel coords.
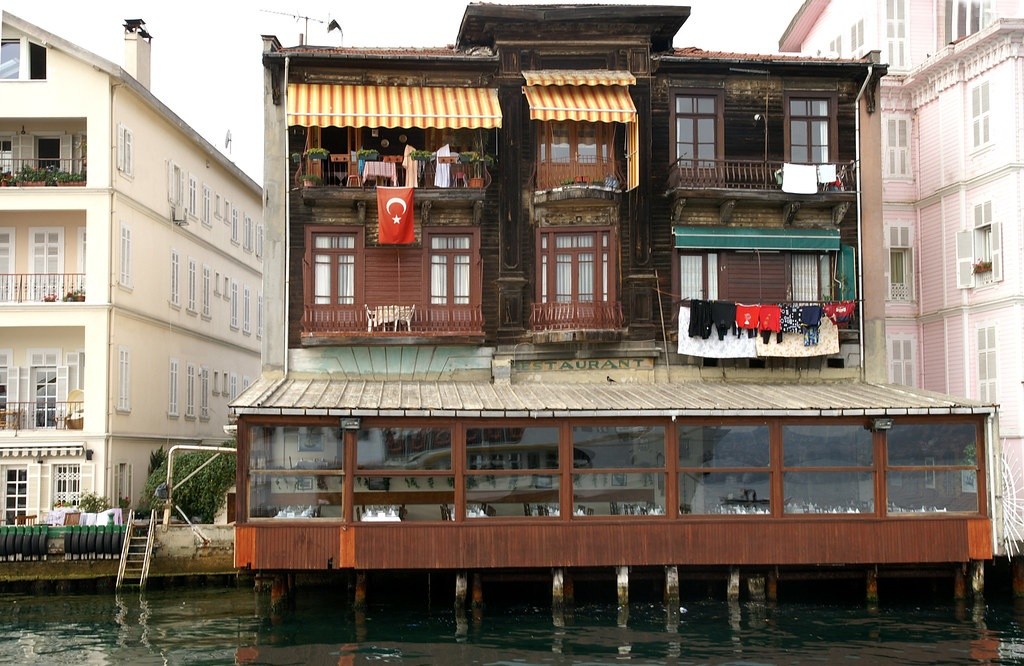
<box><xmin>402</xmin><ymin>144</ymin><xmax>419</xmax><ymax>187</ymax></box>
<box><xmin>432</xmin><ymin>143</ymin><xmax>451</xmax><ymax>189</ymax></box>
<box><xmin>782</xmin><ymin>162</ymin><xmax>838</xmax><ymax>195</ymax></box>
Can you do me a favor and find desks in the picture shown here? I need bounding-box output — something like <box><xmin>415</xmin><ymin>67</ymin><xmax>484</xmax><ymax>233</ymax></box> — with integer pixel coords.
<box><xmin>362</xmin><ymin>161</ymin><xmax>396</xmax><ymax>187</ymax></box>
<box><xmin>361</xmin><ymin>507</ymin><xmax>401</xmax><ymax>521</ymax></box>
<box><xmin>374</xmin><ymin>306</ymin><xmax>409</xmax><ymax>331</ymax></box>
<box><xmin>450</xmin><ymin>507</ymin><xmax>488</xmax><ymax>521</ymax></box>
<box><xmin>531</xmin><ymin>506</ymin><xmax>585</xmax><ymax>516</ymax></box>
<box><xmin>274</xmin><ymin>505</ymin><xmax>316</xmax><ymax>518</ymax></box>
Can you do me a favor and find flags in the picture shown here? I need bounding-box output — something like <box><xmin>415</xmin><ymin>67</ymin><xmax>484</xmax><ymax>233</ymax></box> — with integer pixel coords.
<box><xmin>376</xmin><ymin>186</ymin><xmax>416</xmax><ymax>245</ymax></box>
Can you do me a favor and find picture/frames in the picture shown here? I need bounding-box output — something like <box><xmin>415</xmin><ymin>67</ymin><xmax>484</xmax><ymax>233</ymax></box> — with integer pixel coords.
<box><xmin>535</xmin><ymin>475</ymin><xmax>552</xmax><ymax>489</ymax></box>
<box><xmin>297</xmin><ymin>478</ymin><xmax>318</xmax><ymax>490</ymax></box>
<box><xmin>369</xmin><ymin>477</ymin><xmax>389</xmax><ymax>490</ymax></box>
<box><xmin>298</xmin><ymin>434</ymin><xmax>326</xmax><ymax>452</ymax></box>
<box><xmin>612</xmin><ymin>473</ymin><xmax>627</xmax><ymax>486</ymax></box>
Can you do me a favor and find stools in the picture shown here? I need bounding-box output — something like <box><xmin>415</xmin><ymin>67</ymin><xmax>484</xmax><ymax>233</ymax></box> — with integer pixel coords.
<box><xmin>346</xmin><ymin>175</ymin><xmax>361</xmax><ymax>187</ymax></box>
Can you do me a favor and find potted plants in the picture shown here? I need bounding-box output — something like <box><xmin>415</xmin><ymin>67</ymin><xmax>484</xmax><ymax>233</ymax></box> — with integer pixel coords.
<box><xmin>0</xmin><ymin>165</ymin><xmax>87</xmax><ymax>186</ymax></box>
<box><xmin>972</xmin><ymin>258</ymin><xmax>991</xmax><ymax>275</ymax></box>
<box><xmin>460</xmin><ymin>128</ymin><xmax>494</xmax><ymax>188</ymax></box>
<box><xmin>357</xmin><ymin>149</ymin><xmax>380</xmax><ymax>160</ymax></box>
<box><xmin>54</xmin><ymin>501</ymin><xmax>73</xmax><ymax>507</ymax></box>
<box><xmin>299</xmin><ymin>175</ymin><xmax>324</xmax><ymax>187</ymax></box>
<box><xmin>292</xmin><ymin>153</ymin><xmax>300</xmax><ymax>162</ymax></box>
<box><xmin>77</xmin><ymin>492</ymin><xmax>110</xmax><ymax>526</ymax></box>
<box><xmin>410</xmin><ymin>150</ymin><xmax>432</xmax><ymax>161</ymax></box>
<box><xmin>307</xmin><ymin>148</ymin><xmax>330</xmax><ymax>159</ymax></box>
<box><xmin>560</xmin><ymin>178</ymin><xmax>575</xmax><ymax>184</ymax></box>
<box><xmin>592</xmin><ymin>178</ymin><xmax>604</xmax><ymax>186</ymax></box>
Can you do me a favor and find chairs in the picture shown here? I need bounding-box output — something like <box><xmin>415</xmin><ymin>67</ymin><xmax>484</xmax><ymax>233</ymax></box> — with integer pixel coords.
<box><xmin>312</xmin><ymin>506</ymin><xmax>321</xmax><ymax>518</ymax></box>
<box><xmin>394</xmin><ymin>304</ymin><xmax>415</xmax><ymax>332</ymax></box>
<box><xmin>610</xmin><ymin>500</ymin><xmax>647</xmax><ymax>515</ymax></box>
<box><xmin>66</xmin><ymin>409</ymin><xmax>83</xmax><ymax>429</ymax></box>
<box><xmin>524</xmin><ymin>502</ymin><xmax>549</xmax><ymax>515</ymax></box>
<box><xmin>356</xmin><ymin>503</ymin><xmax>407</xmax><ymax>520</ymax></box>
<box><xmin>709</xmin><ymin>505</ymin><xmax>738</xmax><ymax>515</ymax></box>
<box><xmin>801</xmin><ymin>504</ymin><xmax>830</xmax><ymax>515</ymax></box>
<box><xmin>888</xmin><ymin>500</ymin><xmax>916</xmax><ymax>513</ymax></box>
<box><xmin>578</xmin><ymin>505</ymin><xmax>595</xmax><ymax>516</ymax></box>
<box><xmin>440</xmin><ymin>503</ymin><xmax>450</xmax><ymax>520</ymax></box>
<box><xmin>364</xmin><ymin>304</ymin><xmax>386</xmax><ymax>332</ymax></box>
<box><xmin>271</xmin><ymin>504</ymin><xmax>281</xmax><ymax>517</ymax></box>
<box><xmin>849</xmin><ymin>498</ymin><xmax>871</xmax><ymax>512</ymax></box>
<box><xmin>680</xmin><ymin>503</ymin><xmax>691</xmax><ymax>513</ymax></box>
<box><xmin>0</xmin><ymin>409</ymin><xmax>21</xmax><ymax>428</ymax></box>
<box><xmin>480</xmin><ymin>502</ymin><xmax>496</xmax><ymax>516</ymax></box>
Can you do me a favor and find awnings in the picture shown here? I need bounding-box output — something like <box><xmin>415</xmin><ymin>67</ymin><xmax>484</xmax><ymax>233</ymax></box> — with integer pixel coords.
<box><xmin>520</xmin><ymin>69</ymin><xmax>637</xmax><ymax>123</ymax></box>
<box><xmin>286</xmin><ymin>83</ymin><xmax>503</xmax><ymax>129</ymax></box>
<box><xmin>673</xmin><ymin>225</ymin><xmax>841</xmax><ymax>250</ymax></box>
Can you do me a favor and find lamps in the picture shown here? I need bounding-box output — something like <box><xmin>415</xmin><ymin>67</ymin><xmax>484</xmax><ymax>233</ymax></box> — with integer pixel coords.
<box><xmin>340</xmin><ymin>417</ymin><xmax>360</xmax><ymax>429</ymax></box>
<box><xmin>871</xmin><ymin>419</ymin><xmax>895</xmax><ymax>432</ymax></box>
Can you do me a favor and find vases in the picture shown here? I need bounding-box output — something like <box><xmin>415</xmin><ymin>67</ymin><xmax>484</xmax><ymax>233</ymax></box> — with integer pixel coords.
<box><xmin>76</xmin><ymin>296</ymin><xmax>85</xmax><ymax>301</ymax></box>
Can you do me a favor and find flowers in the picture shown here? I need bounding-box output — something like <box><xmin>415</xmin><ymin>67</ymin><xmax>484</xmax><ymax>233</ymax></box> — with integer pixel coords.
<box><xmin>63</xmin><ymin>289</ymin><xmax>84</xmax><ymax>301</ymax></box>
<box><xmin>43</xmin><ymin>294</ymin><xmax>57</xmax><ymax>301</ymax></box>
